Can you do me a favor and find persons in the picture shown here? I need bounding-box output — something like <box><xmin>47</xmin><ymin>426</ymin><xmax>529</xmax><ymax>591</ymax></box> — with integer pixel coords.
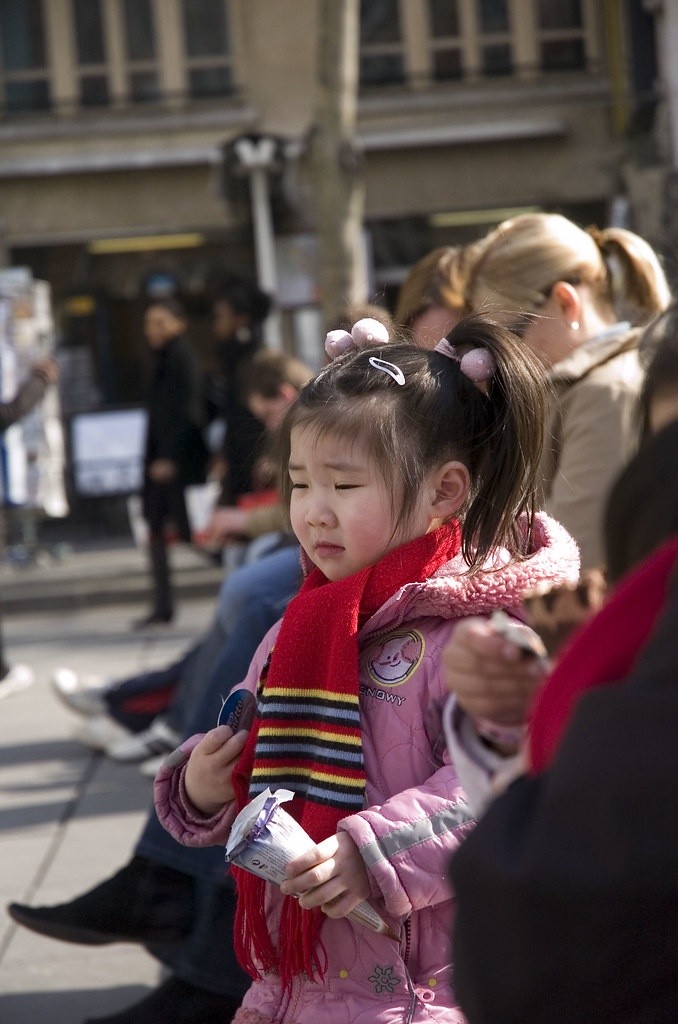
<box><xmin>154</xmin><ymin>313</ymin><xmax>584</xmax><ymax>1024</ymax></box>
<box><xmin>0</xmin><ymin>213</ymin><xmax>678</xmax><ymax>1024</ymax></box>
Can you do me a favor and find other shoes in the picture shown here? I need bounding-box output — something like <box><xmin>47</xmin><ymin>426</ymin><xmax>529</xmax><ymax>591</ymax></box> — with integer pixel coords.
<box><xmin>0</xmin><ymin>665</ymin><xmax>31</xmax><ymax>698</ymax></box>
<box><xmin>134</xmin><ymin>608</ymin><xmax>173</xmax><ymax>630</ymax></box>
<box><xmin>52</xmin><ymin>669</ymin><xmax>111</xmax><ymax>716</ymax></box>
<box><xmin>105</xmin><ymin>720</ymin><xmax>184</xmax><ymax>760</ymax></box>
<box><xmin>71</xmin><ymin>715</ymin><xmax>134</xmax><ymax>750</ymax></box>
<box><xmin>8</xmin><ymin>855</ymin><xmax>204</xmax><ymax>947</ymax></box>
<box><xmin>139</xmin><ymin>754</ymin><xmax>166</xmax><ymax>778</ymax></box>
